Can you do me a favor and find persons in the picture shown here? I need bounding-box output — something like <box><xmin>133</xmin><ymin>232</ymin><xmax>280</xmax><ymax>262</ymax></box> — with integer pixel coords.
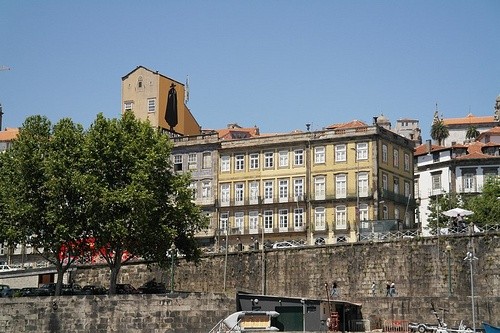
<box><xmin>386</xmin><ymin>282</ymin><xmax>392</xmax><ymax>297</ymax></box>
<box><xmin>390</xmin><ymin>281</ymin><xmax>398</xmax><ymax>297</ymax></box>
<box><xmin>371</xmin><ymin>282</ymin><xmax>376</xmax><ymax>296</ymax></box>
<box><xmin>331</xmin><ymin>282</ymin><xmax>339</xmax><ymax>297</ymax></box>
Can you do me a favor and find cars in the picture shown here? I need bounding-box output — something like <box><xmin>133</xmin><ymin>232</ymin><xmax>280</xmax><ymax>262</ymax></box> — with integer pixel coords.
<box><xmin>0</xmin><ymin>280</ymin><xmax>165</xmax><ymax>297</ymax></box>
<box><xmin>273</xmin><ymin>242</ymin><xmax>303</xmax><ymax>249</ymax></box>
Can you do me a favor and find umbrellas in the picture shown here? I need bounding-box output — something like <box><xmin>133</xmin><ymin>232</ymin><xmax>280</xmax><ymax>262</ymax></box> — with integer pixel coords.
<box><xmin>442</xmin><ymin>208</ymin><xmax>474</xmax><ymax>218</ymax></box>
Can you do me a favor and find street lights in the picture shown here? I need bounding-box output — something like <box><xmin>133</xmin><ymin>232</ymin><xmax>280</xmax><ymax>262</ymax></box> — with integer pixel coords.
<box><xmin>446</xmin><ymin>245</ymin><xmax>452</xmax><ymax>296</ymax></box>
<box><xmin>170</xmin><ymin>242</ymin><xmax>176</xmax><ymax>293</ymax></box>
<box><xmin>257</xmin><ymin>214</ymin><xmax>265</xmax><ymax>294</ymax></box>
